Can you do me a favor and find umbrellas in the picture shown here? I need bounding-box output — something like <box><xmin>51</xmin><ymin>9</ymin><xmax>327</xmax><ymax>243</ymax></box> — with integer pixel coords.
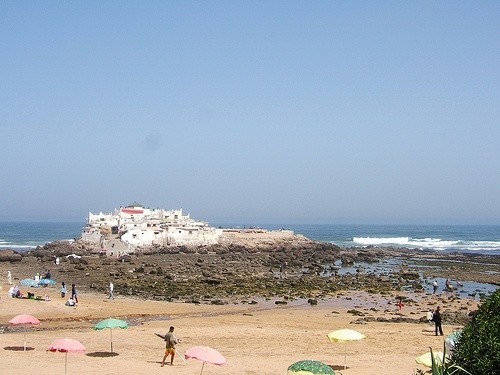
<box><xmin>444</xmin><ymin>331</ymin><xmax>464</xmax><ymax>349</ymax></box>
<box><xmin>37</xmin><ymin>279</ymin><xmax>57</xmax><ymax>293</ymax></box>
<box><xmin>8</xmin><ymin>313</ymin><xmax>128</xmax><ymax>374</ymax></box>
<box><xmin>415</xmin><ymin>351</ymin><xmax>450</xmax><ymax>368</ymax></box>
<box><xmin>326</xmin><ymin>328</ymin><xmax>366</xmax><ymax>370</ymax></box>
<box><xmin>184</xmin><ymin>345</ymin><xmax>227</xmax><ymax>375</ymax></box>
<box><xmin>286</xmin><ymin>360</ymin><xmax>335</xmax><ymax>375</ymax></box>
<box><xmin>18</xmin><ymin>278</ymin><xmax>38</xmax><ymax>292</ymax></box>
<box><xmin>154</xmin><ymin>332</ymin><xmax>187</xmax><ymax>363</ymax></box>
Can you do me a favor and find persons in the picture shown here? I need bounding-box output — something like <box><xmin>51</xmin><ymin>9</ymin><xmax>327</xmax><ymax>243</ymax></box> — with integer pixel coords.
<box><xmin>0</xmin><ymin>256</ymin><xmax>114</xmax><ymax>306</ymax></box>
<box><xmin>432</xmin><ymin>306</ymin><xmax>444</xmax><ymax>336</ymax></box>
<box><xmin>445</xmin><ymin>279</ymin><xmax>452</xmax><ymax>288</ymax></box>
<box><xmin>426</xmin><ymin>309</ymin><xmax>433</xmax><ymax>322</ymax></box>
<box><xmin>161</xmin><ymin>326</ymin><xmax>177</xmax><ymax>367</ymax></box>
<box><xmin>270</xmin><ymin>265</ymin><xmax>283</xmax><ymax>277</ymax></box>
<box><xmin>399</xmin><ymin>299</ymin><xmax>403</xmax><ymax>310</ymax></box>
<box><xmin>433</xmin><ymin>281</ymin><xmax>438</xmax><ymax>294</ymax></box>
<box><xmin>457</xmin><ymin>279</ymin><xmax>461</xmax><ymax>286</ymax></box>
